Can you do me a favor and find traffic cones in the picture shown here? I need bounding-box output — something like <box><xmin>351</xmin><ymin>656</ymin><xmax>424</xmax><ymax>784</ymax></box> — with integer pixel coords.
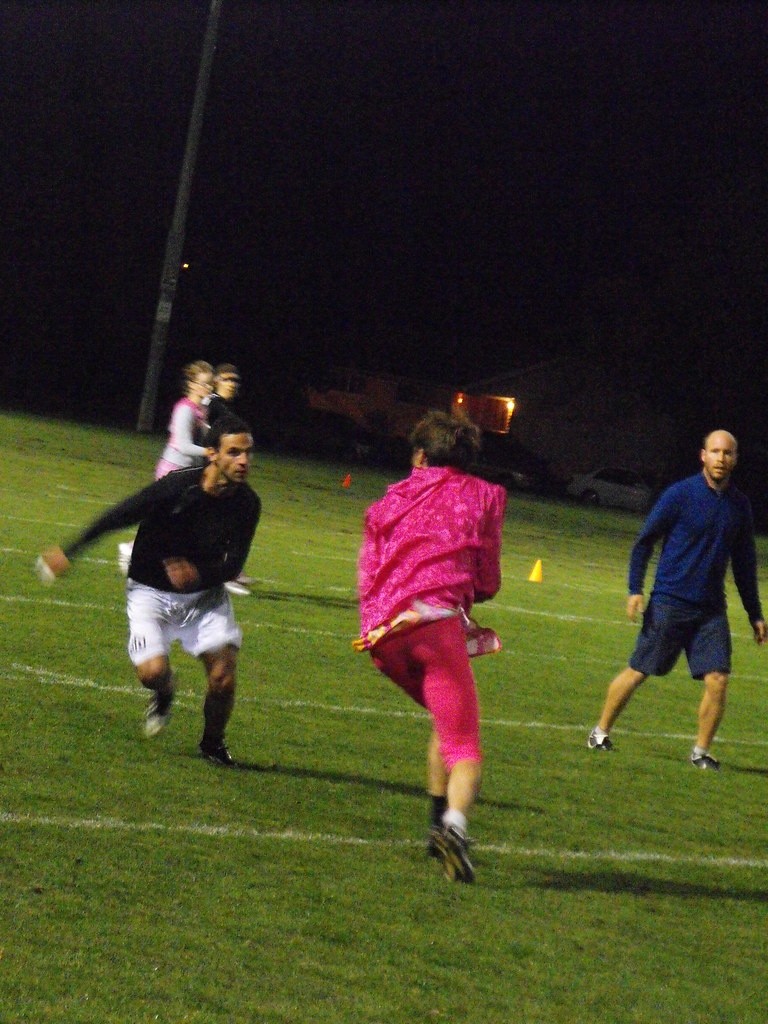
<box><xmin>343</xmin><ymin>474</ymin><xmax>352</xmax><ymax>486</ymax></box>
<box><xmin>527</xmin><ymin>558</ymin><xmax>542</xmax><ymax>582</ymax></box>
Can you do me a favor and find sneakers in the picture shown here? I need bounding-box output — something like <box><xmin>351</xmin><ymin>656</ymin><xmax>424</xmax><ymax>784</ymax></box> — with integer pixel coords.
<box><xmin>142</xmin><ymin>686</ymin><xmax>175</xmax><ymax>736</ymax></box>
<box><xmin>425</xmin><ymin>825</ymin><xmax>476</xmax><ymax>885</ymax></box>
<box><xmin>588</xmin><ymin>730</ymin><xmax>613</xmax><ymax>752</ymax></box>
<box><xmin>199</xmin><ymin>740</ymin><xmax>235</xmax><ymax>768</ymax></box>
<box><xmin>689</xmin><ymin>753</ymin><xmax>719</xmax><ymax>773</ymax></box>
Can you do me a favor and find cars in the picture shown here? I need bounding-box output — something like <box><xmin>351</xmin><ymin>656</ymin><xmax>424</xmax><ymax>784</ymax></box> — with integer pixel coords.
<box><xmin>567</xmin><ymin>466</ymin><xmax>660</xmax><ymax>518</ymax></box>
<box><xmin>469</xmin><ymin>450</ymin><xmax>546</xmax><ymax>494</ymax></box>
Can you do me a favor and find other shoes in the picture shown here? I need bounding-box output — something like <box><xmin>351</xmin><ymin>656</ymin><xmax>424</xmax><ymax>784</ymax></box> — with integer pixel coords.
<box><xmin>223</xmin><ymin>580</ymin><xmax>252</xmax><ymax>596</ymax></box>
<box><xmin>116</xmin><ymin>543</ymin><xmax>134</xmax><ymax>573</ymax></box>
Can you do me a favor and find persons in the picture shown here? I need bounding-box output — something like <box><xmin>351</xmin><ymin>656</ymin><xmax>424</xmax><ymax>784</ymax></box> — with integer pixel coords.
<box><xmin>39</xmin><ymin>415</ymin><xmax>261</xmax><ymax>770</ymax></box>
<box><xmin>586</xmin><ymin>429</ymin><xmax>767</xmax><ymax>770</ymax></box>
<box><xmin>214</xmin><ymin>362</ymin><xmax>258</xmax><ymax>587</ymax></box>
<box><xmin>356</xmin><ymin>411</ymin><xmax>507</xmax><ymax>884</ymax></box>
<box><xmin>117</xmin><ymin>361</ymin><xmax>250</xmax><ymax>596</ymax></box>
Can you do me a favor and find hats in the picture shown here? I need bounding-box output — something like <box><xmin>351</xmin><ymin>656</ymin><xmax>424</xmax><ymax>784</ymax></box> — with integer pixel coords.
<box><xmin>216</xmin><ymin>363</ymin><xmax>241</xmax><ymax>381</ymax></box>
